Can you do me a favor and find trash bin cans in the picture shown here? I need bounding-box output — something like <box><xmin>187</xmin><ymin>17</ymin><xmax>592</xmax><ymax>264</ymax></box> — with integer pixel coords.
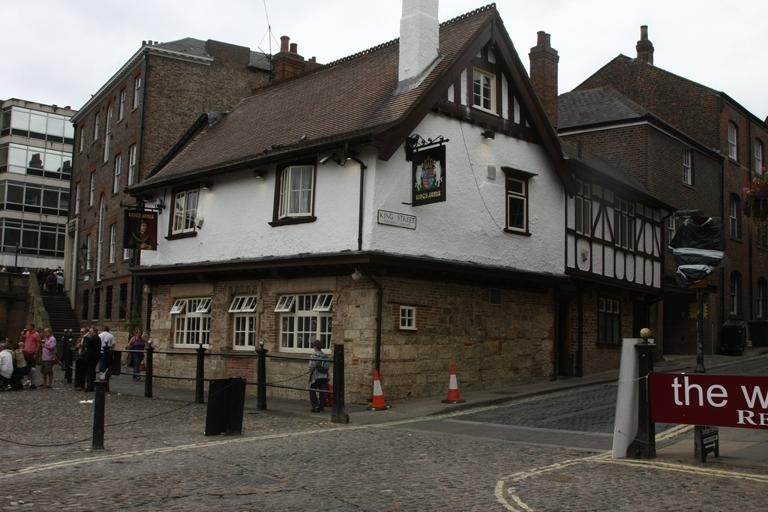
<box><xmin>205</xmin><ymin>378</ymin><xmax>246</xmax><ymax>436</ymax></box>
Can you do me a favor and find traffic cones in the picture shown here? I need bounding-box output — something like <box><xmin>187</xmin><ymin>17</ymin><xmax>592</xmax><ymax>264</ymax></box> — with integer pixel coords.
<box><xmin>441</xmin><ymin>364</ymin><xmax>468</xmax><ymax>404</ymax></box>
<box><xmin>366</xmin><ymin>369</ymin><xmax>391</xmax><ymax>412</ymax></box>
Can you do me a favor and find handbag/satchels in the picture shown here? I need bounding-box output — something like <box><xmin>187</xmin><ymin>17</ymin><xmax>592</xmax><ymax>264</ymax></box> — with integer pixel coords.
<box><xmin>139</xmin><ymin>359</ymin><xmax>145</xmax><ymax>371</ymax></box>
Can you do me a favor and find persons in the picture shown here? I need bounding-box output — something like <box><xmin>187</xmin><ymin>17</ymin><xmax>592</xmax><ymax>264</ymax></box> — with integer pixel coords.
<box><xmin>21</xmin><ymin>266</ymin><xmax>65</xmax><ymax>296</ymax></box>
<box><xmin>307</xmin><ymin>340</ymin><xmax>330</xmax><ymax>414</ymax></box>
<box><xmin>126</xmin><ymin>219</ymin><xmax>155</xmax><ymax>248</ymax></box>
<box><xmin>0</xmin><ymin>266</ymin><xmax>7</xmax><ymax>273</ymax></box>
<box><xmin>0</xmin><ymin>323</ymin><xmax>41</xmax><ymax>391</ymax></box>
<box><xmin>74</xmin><ymin>325</ymin><xmax>115</xmax><ymax>393</ymax></box>
<box><xmin>39</xmin><ymin>327</ymin><xmax>57</xmax><ymax>388</ymax></box>
<box><xmin>126</xmin><ymin>328</ymin><xmax>145</xmax><ymax>382</ymax></box>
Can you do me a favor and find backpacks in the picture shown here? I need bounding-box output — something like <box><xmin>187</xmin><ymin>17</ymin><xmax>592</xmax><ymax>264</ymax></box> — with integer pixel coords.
<box><xmin>315</xmin><ymin>353</ymin><xmax>329</xmax><ymax>374</ymax></box>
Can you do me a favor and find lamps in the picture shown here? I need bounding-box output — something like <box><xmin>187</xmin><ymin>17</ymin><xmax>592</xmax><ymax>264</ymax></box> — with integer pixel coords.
<box><xmin>320</xmin><ymin>152</ymin><xmax>340</xmax><ymax>165</ymax></box>
<box><xmin>481</xmin><ymin>131</ymin><xmax>494</xmax><ymax>139</ymax></box>
<box><xmin>202</xmin><ymin>182</ymin><xmax>213</xmax><ymax>189</ymax></box>
<box><xmin>351</xmin><ymin>267</ymin><xmax>361</xmax><ymax>281</ymax></box>
<box><xmin>255</xmin><ymin>169</ymin><xmax>267</xmax><ymax>178</ymax></box>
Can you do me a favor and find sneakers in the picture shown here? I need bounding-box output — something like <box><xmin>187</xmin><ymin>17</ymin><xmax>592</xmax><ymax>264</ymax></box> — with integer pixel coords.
<box><xmin>24</xmin><ymin>380</ymin><xmax>52</xmax><ymax>388</ymax></box>
